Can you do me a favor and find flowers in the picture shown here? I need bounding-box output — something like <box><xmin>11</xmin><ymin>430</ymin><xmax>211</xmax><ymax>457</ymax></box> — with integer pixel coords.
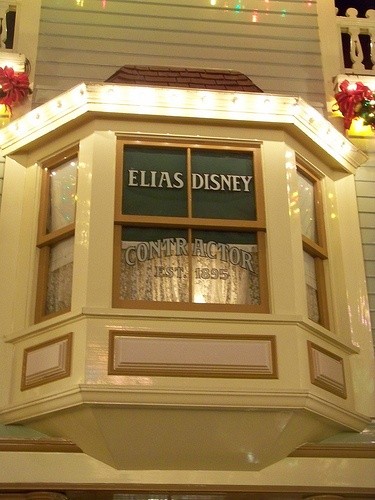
<box><xmin>0</xmin><ymin>64</ymin><xmax>32</xmax><ymax>118</ymax></box>
<box><xmin>335</xmin><ymin>81</ymin><xmax>375</xmax><ymax>133</ymax></box>
